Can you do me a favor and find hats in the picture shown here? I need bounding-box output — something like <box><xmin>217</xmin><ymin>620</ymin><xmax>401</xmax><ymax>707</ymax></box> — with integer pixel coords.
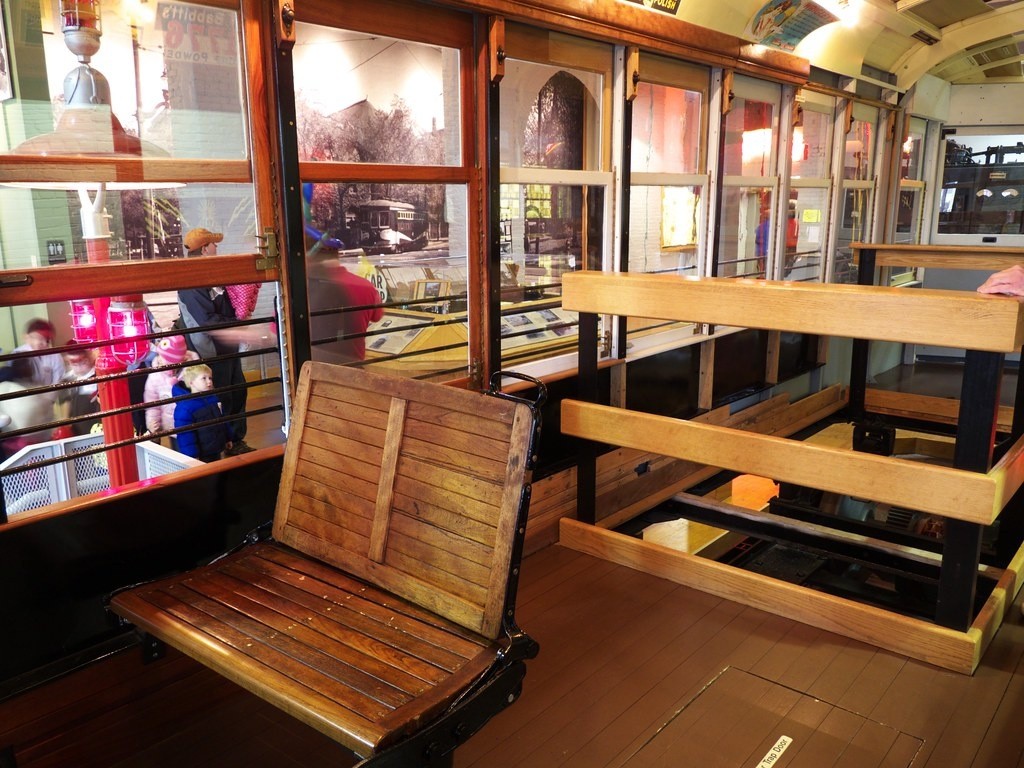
<box><xmin>184</xmin><ymin>228</ymin><xmax>223</xmax><ymax>251</ymax></box>
<box><xmin>156</xmin><ymin>334</ymin><xmax>187</xmax><ymax>364</ymax></box>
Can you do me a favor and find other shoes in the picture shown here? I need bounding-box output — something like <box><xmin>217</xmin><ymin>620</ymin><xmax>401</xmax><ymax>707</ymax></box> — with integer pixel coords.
<box><xmin>224</xmin><ymin>440</ymin><xmax>257</xmax><ymax>457</ymax></box>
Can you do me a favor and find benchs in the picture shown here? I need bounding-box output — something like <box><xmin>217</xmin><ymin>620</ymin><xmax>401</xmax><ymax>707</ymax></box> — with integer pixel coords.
<box><xmin>99</xmin><ymin>357</ymin><xmax>559</xmax><ymax>768</ymax></box>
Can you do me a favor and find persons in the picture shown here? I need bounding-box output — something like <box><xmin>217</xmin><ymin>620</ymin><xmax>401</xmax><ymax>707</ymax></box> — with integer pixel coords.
<box><xmin>977</xmin><ymin>265</ymin><xmax>1024</xmax><ymax>297</ymax></box>
<box><xmin>144</xmin><ymin>336</ymin><xmax>200</xmax><ymax>451</ymax></box>
<box><xmin>172</xmin><ymin>364</ymin><xmax>233</xmax><ymax>463</ymax></box>
<box><xmin>786</xmin><ymin>213</ymin><xmax>798</xmax><ymax>276</ymax></box>
<box><xmin>178</xmin><ymin>228</ymin><xmax>265</xmax><ymax>459</ymax></box>
<box><xmin>270</xmin><ymin>237</ymin><xmax>384</xmax><ymax>371</ymax></box>
<box><xmin>126</xmin><ymin>311</ymin><xmax>163</xmax><ymax>436</ymax></box>
<box><xmin>0</xmin><ymin>320</ymin><xmax>103</xmax><ymax>458</ymax></box>
<box><xmin>755</xmin><ymin>210</ymin><xmax>770</xmax><ymax>269</ymax></box>
